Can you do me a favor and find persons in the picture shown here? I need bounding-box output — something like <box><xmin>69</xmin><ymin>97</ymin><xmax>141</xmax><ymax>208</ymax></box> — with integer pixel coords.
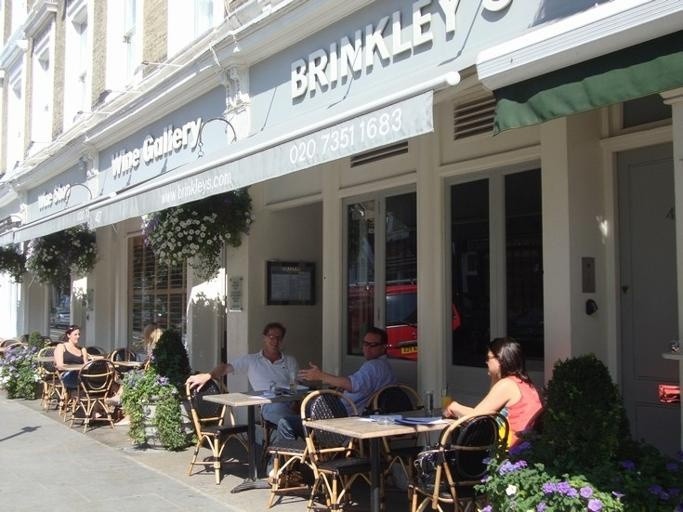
<box><xmin>102</xmin><ymin>321</ymin><xmax>163</xmax><ymax>425</ymax></box>
<box><xmin>442</xmin><ymin>334</ymin><xmax>544</xmax><ymax>455</ymax></box>
<box><xmin>185</xmin><ymin>320</ymin><xmax>301</xmax><ymax>425</ymax></box>
<box><xmin>53</xmin><ymin>324</ymin><xmax>103</xmax><ymax>389</ymax></box>
<box><xmin>266</xmin><ymin>326</ymin><xmax>397</xmax><ymax>480</ymax></box>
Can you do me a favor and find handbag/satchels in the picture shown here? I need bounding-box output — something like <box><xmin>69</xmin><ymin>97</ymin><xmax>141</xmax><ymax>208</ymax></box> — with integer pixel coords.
<box><xmin>413</xmin><ymin>425</ymin><xmax>461</xmax><ymax>494</ymax></box>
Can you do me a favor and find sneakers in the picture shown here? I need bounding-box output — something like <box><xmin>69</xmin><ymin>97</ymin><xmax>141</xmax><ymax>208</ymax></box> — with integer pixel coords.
<box><xmin>105</xmin><ymin>395</ymin><xmax>122</xmax><ymax>407</ymax></box>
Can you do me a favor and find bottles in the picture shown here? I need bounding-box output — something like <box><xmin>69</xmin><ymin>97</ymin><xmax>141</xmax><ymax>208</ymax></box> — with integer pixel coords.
<box><xmin>290</xmin><ymin>372</ymin><xmax>297</xmax><ymax>394</ymax></box>
<box><xmin>424</xmin><ymin>391</ymin><xmax>435</xmax><ymax>417</ymax></box>
<box><xmin>269</xmin><ymin>381</ymin><xmax>275</xmax><ymax>392</ymax></box>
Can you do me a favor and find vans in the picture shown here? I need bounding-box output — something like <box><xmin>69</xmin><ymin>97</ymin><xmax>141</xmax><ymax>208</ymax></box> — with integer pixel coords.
<box><xmin>347</xmin><ymin>281</ymin><xmax>463</xmax><ymax>360</ymax></box>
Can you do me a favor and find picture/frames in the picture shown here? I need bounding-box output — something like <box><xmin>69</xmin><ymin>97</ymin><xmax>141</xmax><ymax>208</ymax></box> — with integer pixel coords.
<box><xmin>266</xmin><ymin>260</ymin><xmax>316</xmax><ymax>305</ymax></box>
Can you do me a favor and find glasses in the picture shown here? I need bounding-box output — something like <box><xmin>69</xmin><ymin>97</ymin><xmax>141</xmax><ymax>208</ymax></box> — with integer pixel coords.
<box><xmin>362</xmin><ymin>340</ymin><xmax>378</xmax><ymax>347</ymax></box>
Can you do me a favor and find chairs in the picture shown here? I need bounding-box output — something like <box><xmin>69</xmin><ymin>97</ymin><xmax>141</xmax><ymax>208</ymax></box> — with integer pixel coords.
<box><xmin>1</xmin><ymin>333</ymin><xmax>144</xmax><ymax>433</ymax></box>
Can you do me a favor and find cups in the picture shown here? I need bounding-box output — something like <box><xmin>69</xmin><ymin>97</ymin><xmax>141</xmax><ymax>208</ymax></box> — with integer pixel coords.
<box><xmin>440</xmin><ymin>387</ymin><xmax>452</xmax><ymax>415</ymax></box>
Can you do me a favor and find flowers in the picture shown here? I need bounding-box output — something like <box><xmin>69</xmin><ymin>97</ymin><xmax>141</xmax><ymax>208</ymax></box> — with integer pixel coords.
<box><xmin>139</xmin><ymin>185</ymin><xmax>256</xmax><ymax>280</ymax></box>
<box><xmin>120</xmin><ymin>365</ymin><xmax>189</xmax><ymax>451</ymax></box>
<box><xmin>0</xmin><ymin>223</ymin><xmax>98</xmax><ymax>299</ymax></box>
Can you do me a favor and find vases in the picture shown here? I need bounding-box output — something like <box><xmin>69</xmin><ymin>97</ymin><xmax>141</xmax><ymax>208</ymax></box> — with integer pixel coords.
<box><xmin>144</xmin><ymin>402</ymin><xmax>192</xmax><ymax>451</ymax></box>
